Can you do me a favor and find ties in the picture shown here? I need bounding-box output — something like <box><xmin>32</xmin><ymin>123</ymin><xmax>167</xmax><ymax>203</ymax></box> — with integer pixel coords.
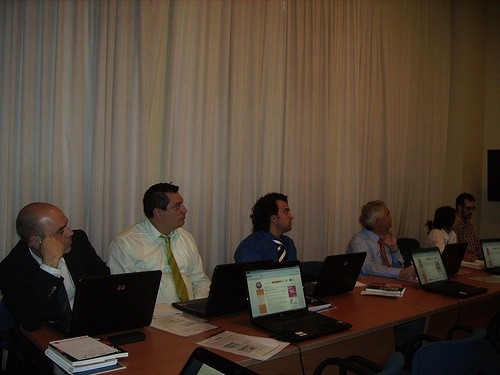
<box><xmin>274</xmin><ymin>239</ymin><xmax>286</xmax><ymax>262</ymax></box>
<box><xmin>160</xmin><ymin>234</ymin><xmax>189</xmax><ymax>301</ymax></box>
<box><xmin>379</xmin><ymin>238</ymin><xmax>390</xmax><ymax>266</ymax></box>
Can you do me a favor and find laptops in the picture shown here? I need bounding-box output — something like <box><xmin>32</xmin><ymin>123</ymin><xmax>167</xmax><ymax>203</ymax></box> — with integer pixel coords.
<box><xmin>179</xmin><ymin>347</ymin><xmax>260</xmax><ymax>375</ymax></box>
<box><xmin>47</xmin><ymin>270</ymin><xmax>162</xmax><ymax>338</ymax></box>
<box><xmin>480</xmin><ymin>238</ymin><xmax>500</xmax><ymax>275</ymax></box>
<box><xmin>171</xmin><ymin>251</ymin><xmax>369</xmax><ymax>344</ymax></box>
<box><xmin>409</xmin><ymin>242</ymin><xmax>488</xmax><ymax>299</ymax></box>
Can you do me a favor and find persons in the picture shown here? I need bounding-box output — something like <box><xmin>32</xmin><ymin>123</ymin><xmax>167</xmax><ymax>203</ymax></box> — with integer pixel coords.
<box><xmin>234</xmin><ymin>192</ymin><xmax>297</xmax><ymax>263</ymax></box>
<box><xmin>347</xmin><ymin>199</ymin><xmax>415</xmax><ymax>279</ymax></box>
<box><xmin>421</xmin><ymin>193</ymin><xmax>480</xmax><ymax>262</ymax></box>
<box><xmin>0</xmin><ymin>202</ymin><xmax>111</xmax><ymax>333</ymax></box>
<box><xmin>107</xmin><ymin>183</ymin><xmax>211</xmax><ymax>305</ymax></box>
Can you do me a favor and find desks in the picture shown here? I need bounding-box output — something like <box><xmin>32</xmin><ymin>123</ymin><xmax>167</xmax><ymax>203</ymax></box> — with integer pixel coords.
<box><xmin>20</xmin><ymin>265</ymin><xmax>500</xmax><ymax>375</ymax></box>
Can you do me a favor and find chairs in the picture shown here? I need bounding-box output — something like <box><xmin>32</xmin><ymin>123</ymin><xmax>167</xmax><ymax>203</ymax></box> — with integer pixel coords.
<box><xmin>313</xmin><ymin>313</ymin><xmax>500</xmax><ymax>375</ymax></box>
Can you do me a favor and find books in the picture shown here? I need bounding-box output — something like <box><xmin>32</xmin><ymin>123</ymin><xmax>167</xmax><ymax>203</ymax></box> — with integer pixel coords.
<box><xmin>307</xmin><ymin>300</ymin><xmax>338</xmax><ymax>314</ymax></box>
<box><xmin>361</xmin><ymin>283</ymin><xmax>406</xmax><ymax>297</ymax></box>
<box><xmin>45</xmin><ymin>335</ymin><xmax>129</xmax><ymax>375</ymax></box>
<box><xmin>461</xmin><ymin>260</ymin><xmax>486</xmax><ymax>270</ymax></box>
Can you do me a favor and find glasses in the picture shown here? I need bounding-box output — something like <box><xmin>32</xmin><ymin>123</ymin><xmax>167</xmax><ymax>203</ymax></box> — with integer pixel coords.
<box><xmin>462</xmin><ymin>207</ymin><xmax>477</xmax><ymax>212</ymax></box>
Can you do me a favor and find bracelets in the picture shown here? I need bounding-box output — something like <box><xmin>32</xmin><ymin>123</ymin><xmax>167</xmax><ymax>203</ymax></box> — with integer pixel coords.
<box><xmin>390</xmin><ymin>247</ymin><xmax>398</xmax><ymax>253</ymax></box>
